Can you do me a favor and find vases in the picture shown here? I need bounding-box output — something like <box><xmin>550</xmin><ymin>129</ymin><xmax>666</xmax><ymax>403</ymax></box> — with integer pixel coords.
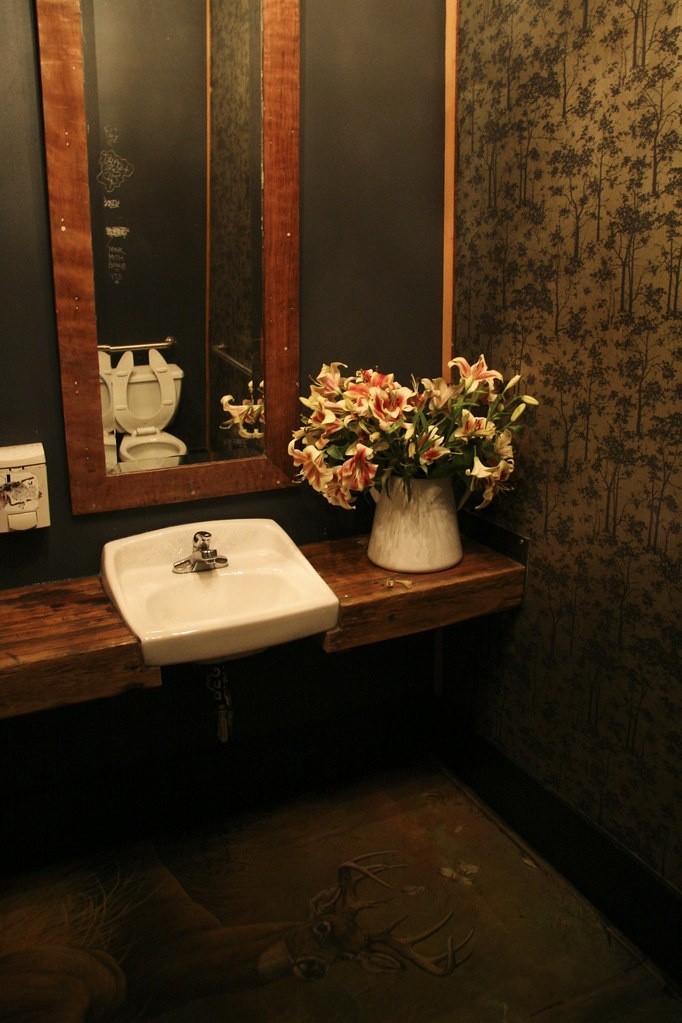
<box><xmin>366</xmin><ymin>474</ymin><xmax>465</xmax><ymax>572</ymax></box>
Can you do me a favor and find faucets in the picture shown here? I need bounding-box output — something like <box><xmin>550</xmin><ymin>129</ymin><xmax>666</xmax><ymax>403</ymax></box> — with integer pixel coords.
<box><xmin>172</xmin><ymin>530</ymin><xmax>230</xmax><ymax>574</ymax></box>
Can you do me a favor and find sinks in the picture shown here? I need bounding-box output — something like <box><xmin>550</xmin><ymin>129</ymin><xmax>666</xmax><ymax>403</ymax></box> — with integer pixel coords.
<box><xmin>100</xmin><ymin>519</ymin><xmax>342</xmax><ymax>667</ymax></box>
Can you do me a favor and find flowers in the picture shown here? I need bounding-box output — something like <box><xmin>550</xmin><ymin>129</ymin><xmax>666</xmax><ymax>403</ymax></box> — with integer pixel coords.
<box><xmin>286</xmin><ymin>355</ymin><xmax>539</xmax><ymax>511</ymax></box>
<box><xmin>219</xmin><ymin>380</ymin><xmax>265</xmax><ymax>439</ymax></box>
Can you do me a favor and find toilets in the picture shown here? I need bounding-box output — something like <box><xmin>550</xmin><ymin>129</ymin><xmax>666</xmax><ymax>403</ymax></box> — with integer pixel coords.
<box><xmin>98</xmin><ymin>347</ymin><xmax>188</xmax><ymax>476</ymax></box>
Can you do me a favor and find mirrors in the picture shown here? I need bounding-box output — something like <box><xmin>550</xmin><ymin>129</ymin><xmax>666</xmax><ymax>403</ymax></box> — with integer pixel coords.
<box><xmin>35</xmin><ymin>0</ymin><xmax>301</xmax><ymax>517</ymax></box>
